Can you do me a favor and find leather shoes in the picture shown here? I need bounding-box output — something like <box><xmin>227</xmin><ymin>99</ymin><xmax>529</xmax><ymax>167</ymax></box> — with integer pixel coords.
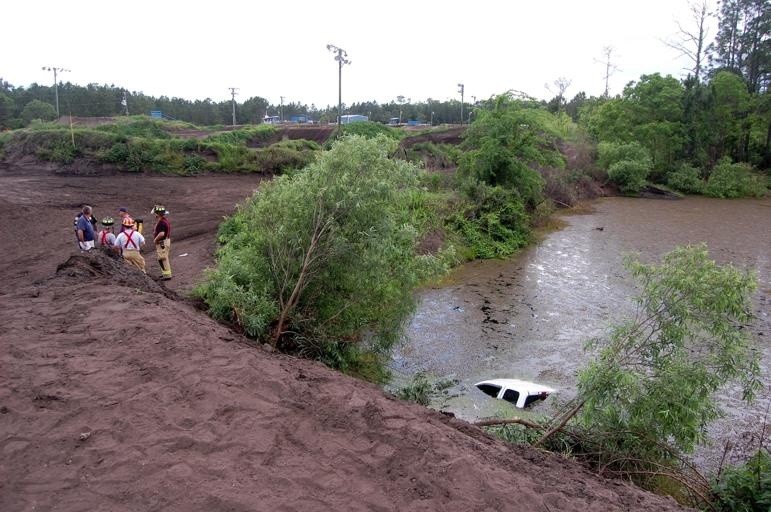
<box><xmin>159</xmin><ymin>274</ymin><xmax>171</xmax><ymax>280</ymax></box>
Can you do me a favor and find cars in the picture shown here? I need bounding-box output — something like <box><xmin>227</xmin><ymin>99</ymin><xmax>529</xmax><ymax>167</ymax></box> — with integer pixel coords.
<box><xmin>475</xmin><ymin>377</ymin><xmax>557</xmax><ymax>408</ymax></box>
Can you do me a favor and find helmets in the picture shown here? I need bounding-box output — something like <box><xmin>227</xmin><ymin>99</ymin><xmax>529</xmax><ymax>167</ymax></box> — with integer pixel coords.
<box><xmin>102</xmin><ymin>217</ymin><xmax>114</xmax><ymax>226</ymax></box>
<box><xmin>151</xmin><ymin>205</ymin><xmax>170</xmax><ymax>215</ymax></box>
<box><xmin>122</xmin><ymin>218</ymin><xmax>134</xmax><ymax>227</ymax></box>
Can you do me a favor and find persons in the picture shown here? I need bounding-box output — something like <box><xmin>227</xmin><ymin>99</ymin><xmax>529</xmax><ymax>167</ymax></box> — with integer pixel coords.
<box><xmin>115</xmin><ymin>217</ymin><xmax>146</xmax><ymax>275</ymax></box>
<box><xmin>98</xmin><ymin>216</ymin><xmax>117</xmax><ymax>251</ymax></box>
<box><xmin>77</xmin><ymin>206</ymin><xmax>95</xmax><ymax>250</ymax></box>
<box><xmin>117</xmin><ymin>207</ymin><xmax>133</xmax><ymax>233</ymax></box>
<box><xmin>74</xmin><ymin>203</ymin><xmax>98</xmax><ymax>245</ymax></box>
<box><xmin>153</xmin><ymin>204</ymin><xmax>173</xmax><ymax>280</ymax></box>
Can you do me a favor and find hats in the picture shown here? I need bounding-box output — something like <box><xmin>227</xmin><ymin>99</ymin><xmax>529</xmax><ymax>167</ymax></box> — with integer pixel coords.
<box><xmin>119</xmin><ymin>207</ymin><xmax>128</xmax><ymax>212</ymax></box>
